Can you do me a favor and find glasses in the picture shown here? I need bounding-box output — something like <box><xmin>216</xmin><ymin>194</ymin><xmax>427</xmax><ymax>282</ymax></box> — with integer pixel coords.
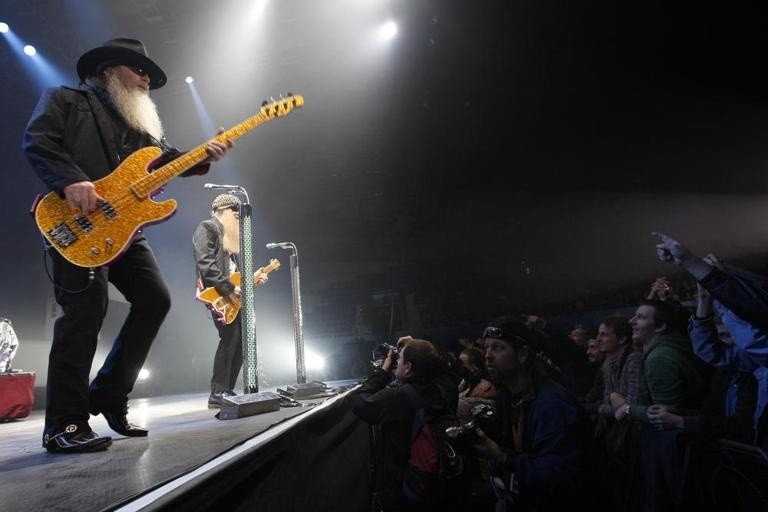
<box><xmin>482</xmin><ymin>327</ymin><xmax>504</xmax><ymax>338</ymax></box>
<box><xmin>127</xmin><ymin>62</ymin><xmax>155</xmax><ymax>80</ymax></box>
<box><xmin>231</xmin><ymin>206</ymin><xmax>239</xmax><ymax>211</ymax></box>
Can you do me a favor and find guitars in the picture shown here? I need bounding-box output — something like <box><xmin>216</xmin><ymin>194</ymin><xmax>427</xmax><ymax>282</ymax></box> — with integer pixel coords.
<box><xmin>195</xmin><ymin>258</ymin><xmax>281</xmax><ymax>325</ymax></box>
<box><xmin>36</xmin><ymin>94</ymin><xmax>303</xmax><ymax>268</ymax></box>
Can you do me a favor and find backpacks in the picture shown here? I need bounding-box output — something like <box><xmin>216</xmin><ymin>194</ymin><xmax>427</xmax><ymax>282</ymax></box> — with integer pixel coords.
<box><xmin>401</xmin><ymin>380</ymin><xmax>468</xmax><ymax>504</ymax></box>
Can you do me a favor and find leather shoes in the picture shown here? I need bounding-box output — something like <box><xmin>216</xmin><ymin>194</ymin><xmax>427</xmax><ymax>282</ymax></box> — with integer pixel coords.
<box><xmin>87</xmin><ymin>393</ymin><xmax>148</xmax><ymax>437</ymax></box>
<box><xmin>208</xmin><ymin>393</ymin><xmax>229</xmax><ymax>409</ymax></box>
<box><xmin>41</xmin><ymin>420</ymin><xmax>113</xmax><ymax>454</ymax></box>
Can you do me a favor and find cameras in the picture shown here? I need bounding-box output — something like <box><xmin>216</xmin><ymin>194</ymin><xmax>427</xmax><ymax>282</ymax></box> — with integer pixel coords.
<box><xmin>379</xmin><ymin>341</ymin><xmax>401</xmax><ymax>359</ymax></box>
<box><xmin>437</xmin><ymin>403</ymin><xmax>510</xmax><ymax>478</ymax></box>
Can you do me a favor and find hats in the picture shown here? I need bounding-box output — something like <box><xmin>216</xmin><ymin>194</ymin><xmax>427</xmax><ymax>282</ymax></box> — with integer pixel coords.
<box><xmin>76</xmin><ymin>37</ymin><xmax>167</xmax><ymax>90</ymax></box>
<box><xmin>211</xmin><ymin>193</ymin><xmax>242</xmax><ymax>210</ymax></box>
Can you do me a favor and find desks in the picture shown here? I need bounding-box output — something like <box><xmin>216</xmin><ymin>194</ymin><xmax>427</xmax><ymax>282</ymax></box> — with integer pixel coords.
<box><xmin>0</xmin><ymin>371</ymin><xmax>36</xmax><ymax>420</ymax></box>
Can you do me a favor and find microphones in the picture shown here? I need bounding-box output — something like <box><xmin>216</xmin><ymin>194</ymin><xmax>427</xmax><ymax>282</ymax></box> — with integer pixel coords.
<box><xmin>266</xmin><ymin>241</ymin><xmax>287</xmax><ymax>249</ymax></box>
<box><xmin>204</xmin><ymin>183</ymin><xmax>239</xmax><ymax>191</ymax></box>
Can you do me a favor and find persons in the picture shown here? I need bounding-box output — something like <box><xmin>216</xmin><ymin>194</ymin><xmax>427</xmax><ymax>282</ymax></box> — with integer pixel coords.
<box><xmin>192</xmin><ymin>192</ymin><xmax>269</xmax><ymax>410</ymax></box>
<box><xmin>23</xmin><ymin>37</ymin><xmax>234</xmax><ymax>455</ymax></box>
<box><xmin>351</xmin><ymin>231</ymin><xmax>768</xmax><ymax>512</ymax></box>
